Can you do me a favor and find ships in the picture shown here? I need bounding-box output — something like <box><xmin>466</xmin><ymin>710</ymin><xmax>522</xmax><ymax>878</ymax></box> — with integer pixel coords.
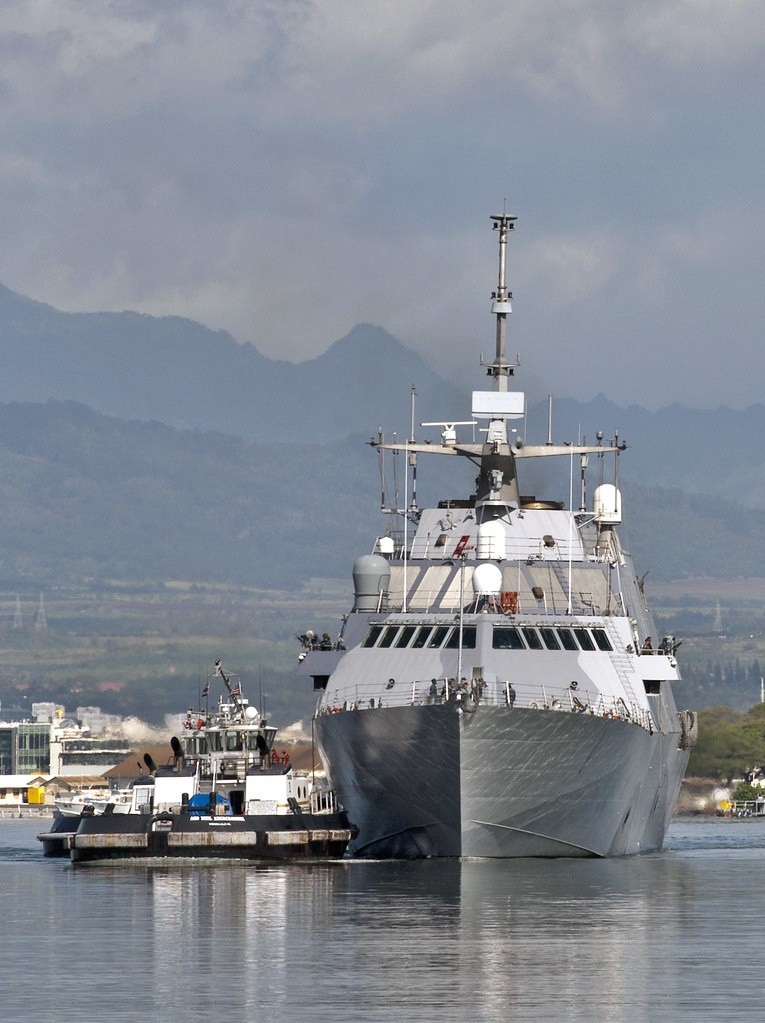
<box><xmin>288</xmin><ymin>200</ymin><xmax>700</xmax><ymax>870</ymax></box>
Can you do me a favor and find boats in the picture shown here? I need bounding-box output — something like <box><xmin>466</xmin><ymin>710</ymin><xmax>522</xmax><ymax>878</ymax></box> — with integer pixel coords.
<box><xmin>731</xmin><ymin>793</ymin><xmax>765</xmax><ymax>819</ymax></box>
<box><xmin>92</xmin><ymin>785</ymin><xmax>135</xmax><ymax>814</ymax></box>
<box><xmin>53</xmin><ymin>784</ymin><xmax>94</xmax><ymax>816</ymax></box>
<box><xmin>35</xmin><ymin>655</ymin><xmax>360</xmax><ymax>867</ymax></box>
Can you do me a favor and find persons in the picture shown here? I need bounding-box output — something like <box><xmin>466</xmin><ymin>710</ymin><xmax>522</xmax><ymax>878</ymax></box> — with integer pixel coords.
<box><xmin>296</xmin><ymin>630</ymin><xmax>347</xmax><ymax>651</ymax></box>
<box><xmin>502</xmin><ymin>682</ymin><xmax>517</xmax><ymax>707</ymax></box>
<box><xmin>271</xmin><ymin>747</ymin><xmax>290</xmax><ymax>764</ymax></box>
<box><xmin>642</xmin><ymin>634</ymin><xmax>685</xmax><ymax>657</ymax></box>
<box><xmin>426</xmin><ymin>677</ymin><xmax>486</xmax><ymax>706</ymax></box>
